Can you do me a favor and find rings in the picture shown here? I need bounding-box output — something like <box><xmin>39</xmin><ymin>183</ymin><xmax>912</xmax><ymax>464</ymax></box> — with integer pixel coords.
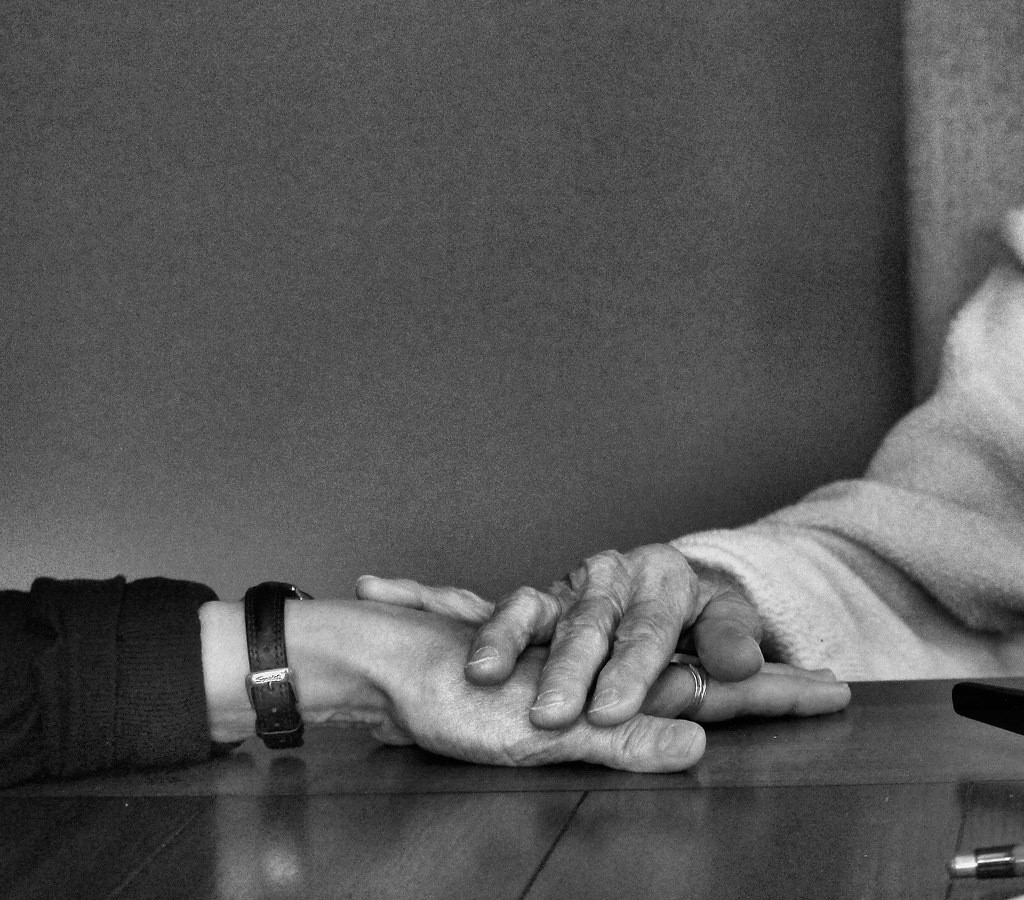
<box><xmin>668</xmin><ymin>653</ymin><xmax>711</xmax><ymax>722</ymax></box>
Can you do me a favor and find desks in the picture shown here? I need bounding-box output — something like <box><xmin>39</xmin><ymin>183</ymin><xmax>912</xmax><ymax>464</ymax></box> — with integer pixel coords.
<box><xmin>0</xmin><ymin>678</ymin><xmax>1024</xmax><ymax>900</ymax></box>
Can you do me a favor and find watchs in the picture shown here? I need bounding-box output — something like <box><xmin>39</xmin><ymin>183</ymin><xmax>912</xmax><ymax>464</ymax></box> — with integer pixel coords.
<box><xmin>239</xmin><ymin>581</ymin><xmax>315</xmax><ymax>751</ymax></box>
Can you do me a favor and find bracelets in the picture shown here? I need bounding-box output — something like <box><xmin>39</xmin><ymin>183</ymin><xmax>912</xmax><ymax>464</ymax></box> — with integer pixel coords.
<box><xmin>0</xmin><ymin>572</ymin><xmax>854</xmax><ymax>797</ymax></box>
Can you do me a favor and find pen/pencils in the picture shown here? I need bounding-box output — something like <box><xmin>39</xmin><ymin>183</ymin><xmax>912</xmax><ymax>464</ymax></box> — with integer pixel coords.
<box><xmin>946</xmin><ymin>843</ymin><xmax>1024</xmax><ymax>878</ymax></box>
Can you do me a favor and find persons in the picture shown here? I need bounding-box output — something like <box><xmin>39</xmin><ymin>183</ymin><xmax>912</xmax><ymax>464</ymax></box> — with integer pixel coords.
<box><xmin>350</xmin><ymin>172</ymin><xmax>1024</xmax><ymax>733</ymax></box>
